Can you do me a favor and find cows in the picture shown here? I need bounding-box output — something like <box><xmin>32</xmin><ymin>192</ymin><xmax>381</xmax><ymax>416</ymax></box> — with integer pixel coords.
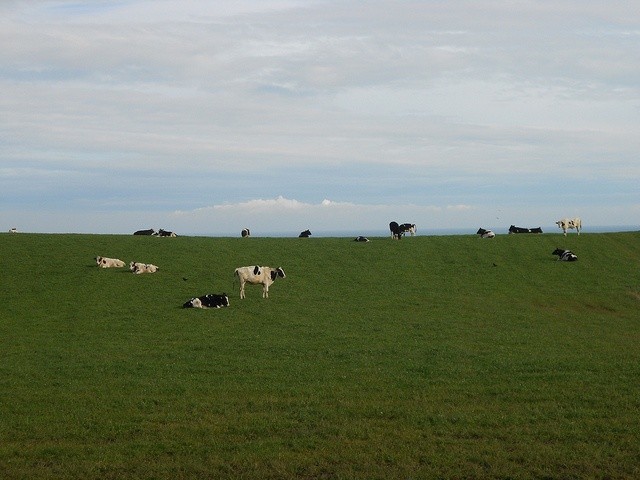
<box><xmin>529</xmin><ymin>227</ymin><xmax>544</xmax><ymax>234</ymax></box>
<box><xmin>389</xmin><ymin>221</ymin><xmax>403</xmax><ymax>240</ymax></box>
<box><xmin>508</xmin><ymin>225</ymin><xmax>529</xmax><ymax>234</ymax></box>
<box><xmin>157</xmin><ymin>229</ymin><xmax>178</xmax><ymax>237</ymax></box>
<box><xmin>134</xmin><ymin>229</ymin><xmax>155</xmax><ymax>235</ymax></box>
<box><xmin>551</xmin><ymin>248</ymin><xmax>579</xmax><ymax>262</ymax></box>
<box><xmin>94</xmin><ymin>256</ymin><xmax>126</xmax><ymax>268</ymax></box>
<box><xmin>477</xmin><ymin>227</ymin><xmax>497</xmax><ymax>239</ymax></box>
<box><xmin>129</xmin><ymin>261</ymin><xmax>160</xmax><ymax>274</ymax></box>
<box><xmin>299</xmin><ymin>229</ymin><xmax>312</xmax><ymax>238</ymax></box>
<box><xmin>233</xmin><ymin>265</ymin><xmax>286</xmax><ymax>299</ymax></box>
<box><xmin>354</xmin><ymin>236</ymin><xmax>370</xmax><ymax>243</ymax></box>
<box><xmin>398</xmin><ymin>223</ymin><xmax>418</xmax><ymax>236</ymax></box>
<box><xmin>241</xmin><ymin>228</ymin><xmax>251</xmax><ymax>238</ymax></box>
<box><xmin>182</xmin><ymin>292</ymin><xmax>230</xmax><ymax>310</ymax></box>
<box><xmin>556</xmin><ymin>217</ymin><xmax>583</xmax><ymax>236</ymax></box>
